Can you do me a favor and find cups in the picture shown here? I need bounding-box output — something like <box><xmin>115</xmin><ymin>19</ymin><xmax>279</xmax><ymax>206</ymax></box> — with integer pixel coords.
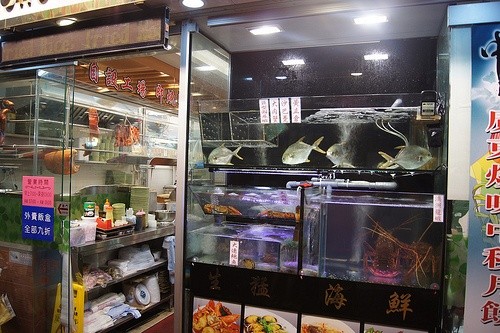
<box><xmin>84</xmin><ymin>134</ymin><xmax>120</xmax><ymax>162</ymax></box>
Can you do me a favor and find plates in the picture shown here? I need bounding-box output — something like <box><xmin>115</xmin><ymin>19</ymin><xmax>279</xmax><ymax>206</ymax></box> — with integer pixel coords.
<box><xmin>192</xmin><ymin>305</ymin><xmax>236</xmax><ymax>332</ymax></box>
<box><xmin>301</xmin><ymin>315</ymin><xmax>355</xmax><ymax>333</ymax></box>
<box><xmin>244</xmin><ymin>308</ymin><xmax>297</xmax><ymax>333</ymax></box>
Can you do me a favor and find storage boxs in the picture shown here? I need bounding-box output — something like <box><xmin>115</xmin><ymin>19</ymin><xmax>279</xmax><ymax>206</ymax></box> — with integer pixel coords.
<box><xmin>197</xmin><ymin>91</ymin><xmax>447</xmax><ymax>189</ymax></box>
<box><xmin>186</xmin><ymin>182</ymin><xmax>320</xmax><ymax>276</ymax></box>
<box><xmin>301</xmin><ymin>189</ymin><xmax>443</xmax><ymax>290</ymax></box>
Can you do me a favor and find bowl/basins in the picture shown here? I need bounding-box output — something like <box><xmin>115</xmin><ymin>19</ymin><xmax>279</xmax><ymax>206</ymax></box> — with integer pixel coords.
<box><xmin>149</xmin><ymin>201</ymin><xmax>177</xmax><ymax>222</ymax></box>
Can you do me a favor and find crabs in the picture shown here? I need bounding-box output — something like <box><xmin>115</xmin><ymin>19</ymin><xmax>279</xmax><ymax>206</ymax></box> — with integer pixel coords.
<box><xmin>204</xmin><ymin>204</ymin><xmax>242</xmax><ymax>215</ymax></box>
<box><xmin>256</xmin><ymin>210</ymin><xmax>295</xmax><ymax>219</ymax></box>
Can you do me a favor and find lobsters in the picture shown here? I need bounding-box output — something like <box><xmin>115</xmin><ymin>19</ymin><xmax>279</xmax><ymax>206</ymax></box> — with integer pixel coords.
<box><xmin>361</xmin><ymin>212</ymin><xmax>436</xmax><ymax>288</ymax></box>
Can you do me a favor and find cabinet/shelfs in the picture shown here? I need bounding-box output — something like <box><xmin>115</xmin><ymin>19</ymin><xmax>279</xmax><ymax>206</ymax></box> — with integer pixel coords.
<box><xmin>71</xmin><ymin>224</ymin><xmax>174</xmax><ymax>332</ymax></box>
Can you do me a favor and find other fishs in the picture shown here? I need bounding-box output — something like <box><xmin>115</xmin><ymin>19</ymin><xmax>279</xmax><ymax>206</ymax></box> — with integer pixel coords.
<box><xmin>324</xmin><ymin>143</ymin><xmax>357</xmax><ymax>168</ymax></box>
<box><xmin>377</xmin><ymin>145</ymin><xmax>432</xmax><ymax>170</ymax></box>
<box><xmin>208</xmin><ymin>143</ymin><xmax>243</xmax><ymax>166</ymax></box>
<box><xmin>281</xmin><ymin>135</ymin><xmax>327</xmax><ymax>166</ymax></box>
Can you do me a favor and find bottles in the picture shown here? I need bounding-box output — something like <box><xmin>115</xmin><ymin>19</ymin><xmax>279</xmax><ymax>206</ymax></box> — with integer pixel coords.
<box><xmin>70</xmin><ymin>198</ymin><xmax>146</xmax><ymax>243</ymax></box>
